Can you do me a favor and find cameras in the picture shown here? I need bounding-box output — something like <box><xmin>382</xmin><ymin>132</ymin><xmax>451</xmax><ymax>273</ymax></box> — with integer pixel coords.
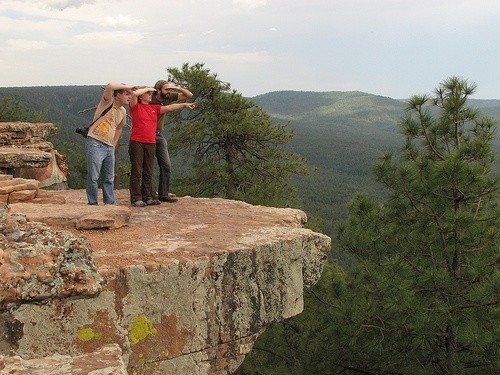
<box><xmin>75</xmin><ymin>127</ymin><xmax>88</xmax><ymax>137</ymax></box>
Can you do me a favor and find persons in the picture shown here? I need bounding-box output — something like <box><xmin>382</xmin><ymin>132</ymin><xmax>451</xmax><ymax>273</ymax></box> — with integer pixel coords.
<box><xmin>86</xmin><ymin>84</ymin><xmax>137</xmax><ymax>205</ymax></box>
<box><xmin>149</xmin><ymin>80</ymin><xmax>193</xmax><ymax>204</ymax></box>
<box><xmin>129</xmin><ymin>86</ymin><xmax>198</xmax><ymax>207</ymax></box>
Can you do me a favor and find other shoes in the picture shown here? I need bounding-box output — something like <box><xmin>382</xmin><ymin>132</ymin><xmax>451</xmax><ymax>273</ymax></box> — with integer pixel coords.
<box><xmin>131</xmin><ymin>201</ymin><xmax>146</xmax><ymax>207</ymax></box>
<box><xmin>146</xmin><ymin>199</ymin><xmax>161</xmax><ymax>205</ymax></box>
<box><xmin>160</xmin><ymin>197</ymin><xmax>177</xmax><ymax>202</ymax></box>
